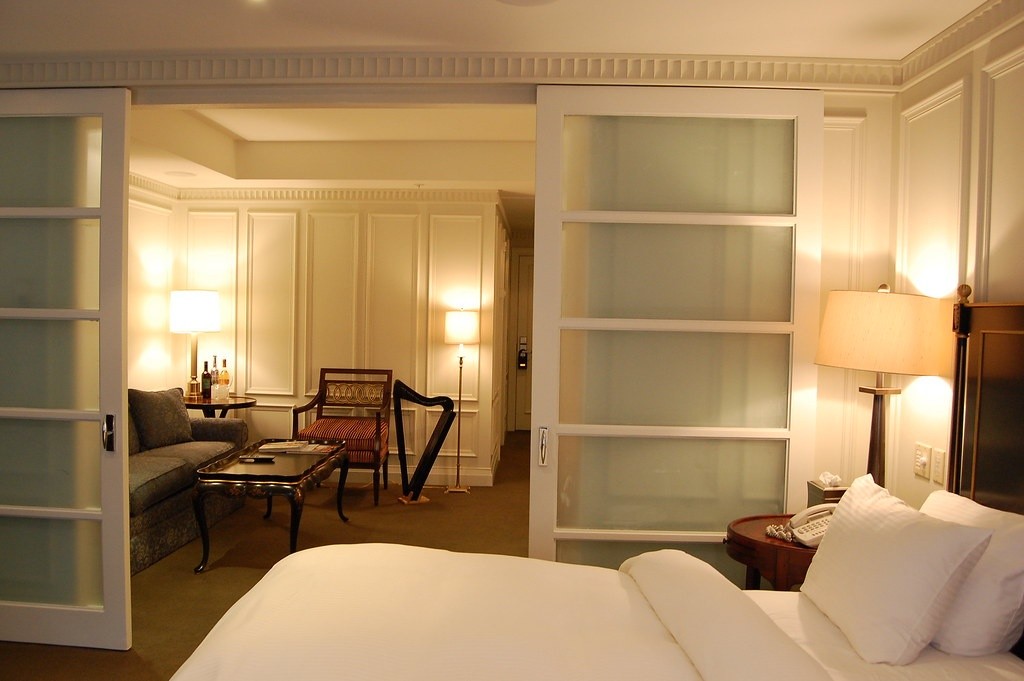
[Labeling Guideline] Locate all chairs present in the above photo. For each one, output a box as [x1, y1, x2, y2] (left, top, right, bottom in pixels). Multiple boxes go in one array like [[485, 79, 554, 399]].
[[293, 367, 393, 506]]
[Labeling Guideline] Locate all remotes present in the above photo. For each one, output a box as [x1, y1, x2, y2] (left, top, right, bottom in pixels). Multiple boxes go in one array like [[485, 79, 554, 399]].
[[239, 454, 275, 463]]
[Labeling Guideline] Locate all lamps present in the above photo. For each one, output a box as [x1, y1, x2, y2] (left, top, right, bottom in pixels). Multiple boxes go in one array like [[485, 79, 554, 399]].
[[444, 308, 479, 495], [813, 284, 957, 491], [168, 289, 221, 397]]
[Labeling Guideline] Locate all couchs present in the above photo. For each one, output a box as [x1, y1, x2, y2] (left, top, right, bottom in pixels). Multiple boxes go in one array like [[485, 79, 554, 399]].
[[128, 404, 248, 577]]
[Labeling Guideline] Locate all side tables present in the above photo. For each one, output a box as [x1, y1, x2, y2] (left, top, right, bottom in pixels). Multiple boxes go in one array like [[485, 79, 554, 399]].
[[182, 396, 257, 418]]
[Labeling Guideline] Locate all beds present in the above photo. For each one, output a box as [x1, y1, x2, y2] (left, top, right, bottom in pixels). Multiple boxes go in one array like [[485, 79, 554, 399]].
[[169, 286, 1024, 680]]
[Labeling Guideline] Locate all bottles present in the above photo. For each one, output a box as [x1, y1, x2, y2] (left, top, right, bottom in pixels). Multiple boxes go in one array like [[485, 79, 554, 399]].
[[201, 361, 212, 399], [219, 359, 230, 385], [211, 356, 220, 385]]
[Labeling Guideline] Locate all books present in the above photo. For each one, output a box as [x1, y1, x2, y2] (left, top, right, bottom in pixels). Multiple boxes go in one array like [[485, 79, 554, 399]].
[[258, 441, 309, 452], [285, 442, 338, 456]]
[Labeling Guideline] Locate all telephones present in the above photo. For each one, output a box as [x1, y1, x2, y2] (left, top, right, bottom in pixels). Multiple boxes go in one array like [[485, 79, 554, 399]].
[[766, 502, 840, 548]]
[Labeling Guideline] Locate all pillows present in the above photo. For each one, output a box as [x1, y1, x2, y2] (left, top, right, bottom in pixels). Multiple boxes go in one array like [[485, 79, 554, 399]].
[[799, 473, 994, 666], [128, 387, 194, 447], [918, 490, 1024, 655]]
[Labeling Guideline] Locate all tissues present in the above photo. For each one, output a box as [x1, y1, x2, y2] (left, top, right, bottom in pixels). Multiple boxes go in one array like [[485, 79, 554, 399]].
[[807, 468, 849, 508]]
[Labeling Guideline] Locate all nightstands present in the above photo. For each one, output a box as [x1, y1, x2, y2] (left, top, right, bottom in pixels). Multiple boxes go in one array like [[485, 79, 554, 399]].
[[722, 513, 817, 590]]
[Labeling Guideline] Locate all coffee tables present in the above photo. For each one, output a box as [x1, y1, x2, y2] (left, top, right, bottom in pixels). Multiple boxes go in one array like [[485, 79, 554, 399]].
[[191, 438, 350, 577]]
